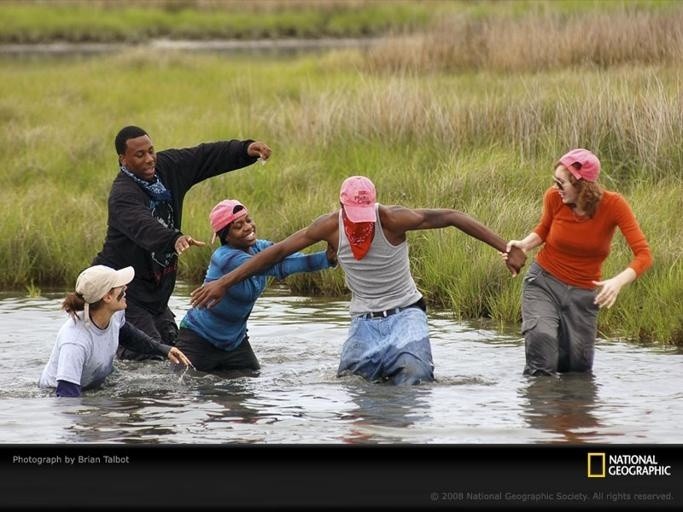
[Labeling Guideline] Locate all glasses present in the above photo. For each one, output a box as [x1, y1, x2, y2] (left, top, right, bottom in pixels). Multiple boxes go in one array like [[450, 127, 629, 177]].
[[552, 177, 573, 191]]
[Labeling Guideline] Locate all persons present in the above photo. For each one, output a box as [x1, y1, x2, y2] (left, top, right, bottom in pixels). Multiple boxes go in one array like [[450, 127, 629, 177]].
[[161, 200, 341, 382], [496, 148, 653, 374], [190, 176, 527, 386], [87, 125, 271, 358], [34, 265, 193, 396]]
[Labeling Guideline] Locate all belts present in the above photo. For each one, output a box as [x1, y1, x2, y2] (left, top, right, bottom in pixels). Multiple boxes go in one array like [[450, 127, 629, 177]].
[[357, 307, 405, 319]]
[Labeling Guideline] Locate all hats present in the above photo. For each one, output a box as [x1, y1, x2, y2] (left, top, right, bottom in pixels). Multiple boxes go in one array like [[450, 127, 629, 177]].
[[208, 199, 250, 245], [559, 148, 601, 183], [75, 265, 136, 304], [340, 176, 377, 223]]
